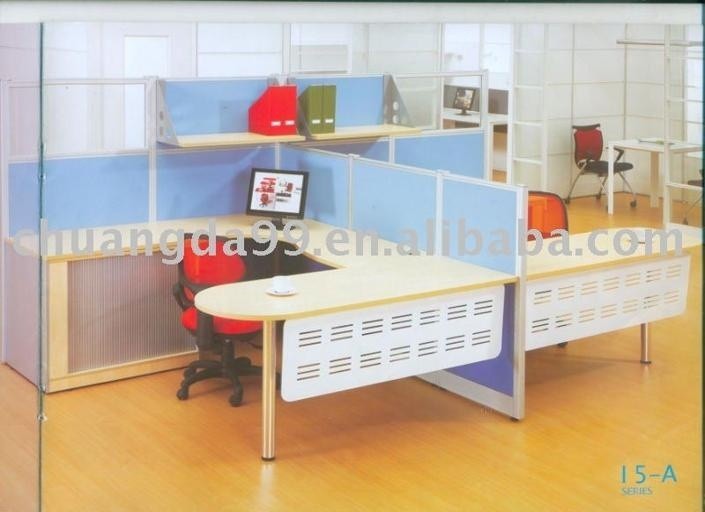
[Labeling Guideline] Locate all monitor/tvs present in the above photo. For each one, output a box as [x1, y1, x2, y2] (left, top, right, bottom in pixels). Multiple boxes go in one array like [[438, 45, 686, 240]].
[[452, 87, 476, 116], [245, 167, 309, 230]]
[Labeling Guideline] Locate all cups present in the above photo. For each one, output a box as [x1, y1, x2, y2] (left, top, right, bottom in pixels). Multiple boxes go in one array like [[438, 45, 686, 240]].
[[271, 275, 291, 292]]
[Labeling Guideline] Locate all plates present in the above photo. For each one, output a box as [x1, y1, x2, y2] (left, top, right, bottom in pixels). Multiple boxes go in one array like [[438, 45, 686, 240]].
[[266, 288, 298, 296]]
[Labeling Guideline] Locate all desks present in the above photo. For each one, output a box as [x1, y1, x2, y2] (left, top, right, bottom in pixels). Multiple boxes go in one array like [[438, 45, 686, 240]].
[[5, 213, 518, 461], [526, 223, 703, 364], [607, 139, 703, 216], [444, 108, 508, 128]]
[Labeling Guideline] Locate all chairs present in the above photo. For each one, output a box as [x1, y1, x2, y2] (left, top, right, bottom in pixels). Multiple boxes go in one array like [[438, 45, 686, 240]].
[[172, 233, 281, 408], [528, 190, 568, 348], [565, 123, 637, 212]]
